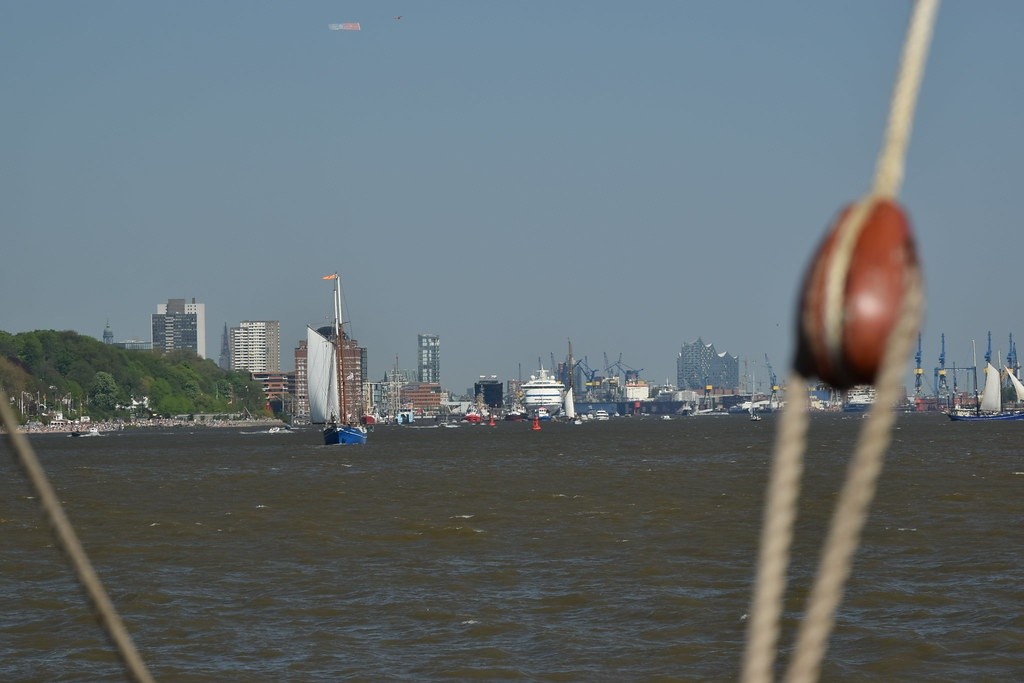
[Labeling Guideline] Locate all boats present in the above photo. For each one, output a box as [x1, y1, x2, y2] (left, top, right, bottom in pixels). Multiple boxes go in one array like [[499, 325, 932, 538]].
[[519, 369, 565, 417], [588, 414, 593, 420], [89, 428, 97, 435], [652, 376, 699, 414], [533, 408, 551, 422], [844, 395, 875, 412], [270, 427, 288, 433], [596, 410, 609, 421], [360, 407, 379, 425], [465, 408, 484, 423], [662, 416, 671, 420], [71, 432, 81, 435], [581, 415, 588, 421], [505, 407, 529, 423], [575, 419, 581, 424]]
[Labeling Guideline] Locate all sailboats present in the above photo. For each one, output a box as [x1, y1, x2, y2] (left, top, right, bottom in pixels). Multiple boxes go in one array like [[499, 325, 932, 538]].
[[321, 270, 367, 447], [946, 350, 1024, 421], [749, 361, 761, 421]]
